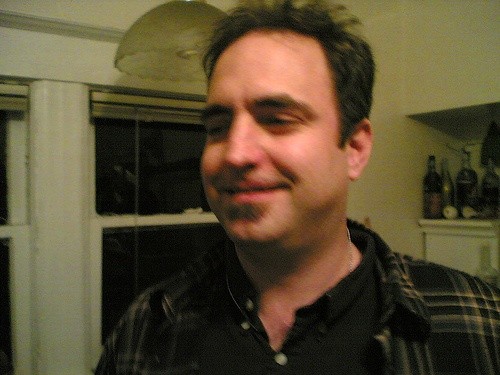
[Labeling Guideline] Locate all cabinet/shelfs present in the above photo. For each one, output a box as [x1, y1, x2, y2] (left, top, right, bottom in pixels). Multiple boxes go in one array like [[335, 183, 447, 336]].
[[418, 219, 500, 275]]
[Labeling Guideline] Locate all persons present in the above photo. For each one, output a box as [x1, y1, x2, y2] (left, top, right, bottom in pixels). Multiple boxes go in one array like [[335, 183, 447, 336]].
[[93, 0, 500, 375]]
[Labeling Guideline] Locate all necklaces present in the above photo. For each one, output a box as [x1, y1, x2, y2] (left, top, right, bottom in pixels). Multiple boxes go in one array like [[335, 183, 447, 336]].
[[345, 226, 354, 277]]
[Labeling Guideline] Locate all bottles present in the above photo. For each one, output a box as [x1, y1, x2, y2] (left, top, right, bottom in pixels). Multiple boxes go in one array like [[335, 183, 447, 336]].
[[422, 155, 442, 220], [442, 158, 458, 220], [476, 245, 500, 289], [479, 156, 500, 219], [455, 151, 479, 220]]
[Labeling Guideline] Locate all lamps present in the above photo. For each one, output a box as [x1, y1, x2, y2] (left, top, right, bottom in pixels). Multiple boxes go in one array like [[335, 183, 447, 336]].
[[113, 0, 228, 82]]
